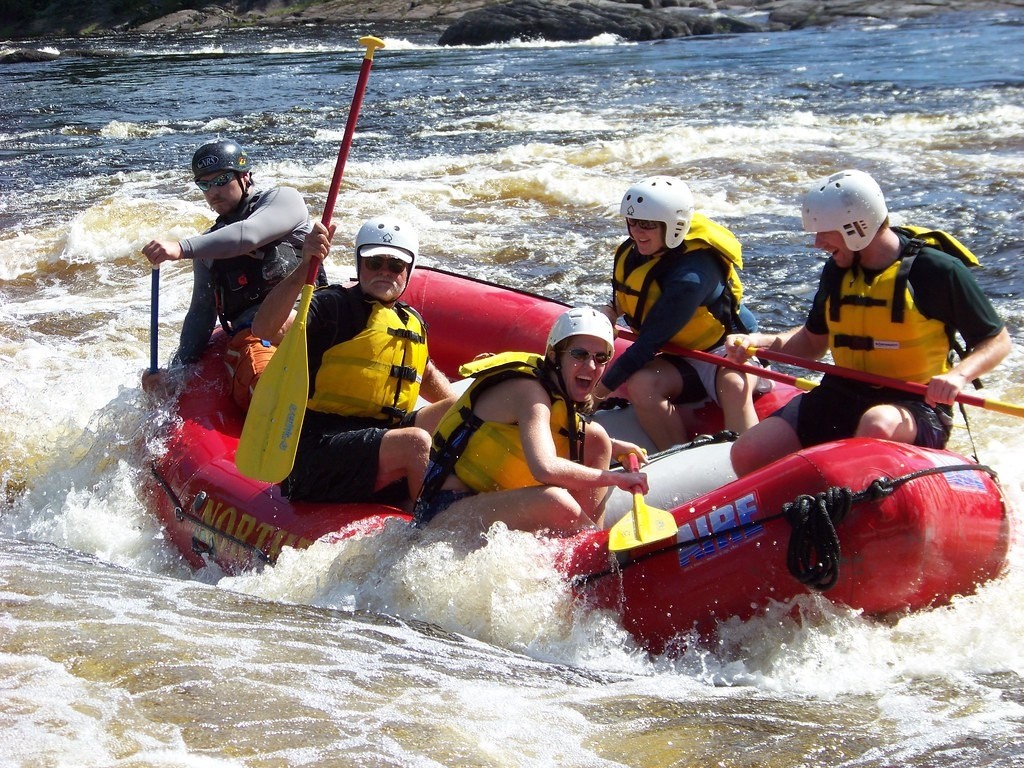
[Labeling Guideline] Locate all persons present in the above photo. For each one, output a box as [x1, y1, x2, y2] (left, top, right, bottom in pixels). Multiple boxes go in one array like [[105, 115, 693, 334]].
[[586, 175, 774, 451], [250, 215, 459, 512], [723, 169, 1012, 479], [141, 141, 329, 412], [412, 307, 647, 538]]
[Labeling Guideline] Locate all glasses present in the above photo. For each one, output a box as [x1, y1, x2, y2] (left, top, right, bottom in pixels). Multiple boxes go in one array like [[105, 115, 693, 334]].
[[628, 217, 660, 230], [362, 255, 406, 274], [196, 170, 235, 191], [557, 348, 612, 365]]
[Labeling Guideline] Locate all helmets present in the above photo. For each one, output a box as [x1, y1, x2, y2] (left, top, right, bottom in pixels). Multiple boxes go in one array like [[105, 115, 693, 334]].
[[619, 175, 694, 249], [802, 169, 889, 252], [355, 214, 419, 286], [545, 307, 615, 365], [191, 141, 252, 182]]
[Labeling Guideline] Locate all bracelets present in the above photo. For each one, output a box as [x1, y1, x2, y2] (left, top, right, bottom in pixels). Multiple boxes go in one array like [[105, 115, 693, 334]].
[[593, 394, 605, 401]]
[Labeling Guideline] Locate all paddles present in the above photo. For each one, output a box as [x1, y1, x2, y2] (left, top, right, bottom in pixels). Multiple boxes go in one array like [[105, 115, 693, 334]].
[[149, 261, 160, 374], [607, 447, 680, 552], [614, 327, 972, 429], [234, 34, 386, 483], [734, 338, 1024, 419]]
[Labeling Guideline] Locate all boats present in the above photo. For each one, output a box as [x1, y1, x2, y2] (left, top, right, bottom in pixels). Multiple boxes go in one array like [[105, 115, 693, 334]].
[[134, 266, 1013, 658]]
